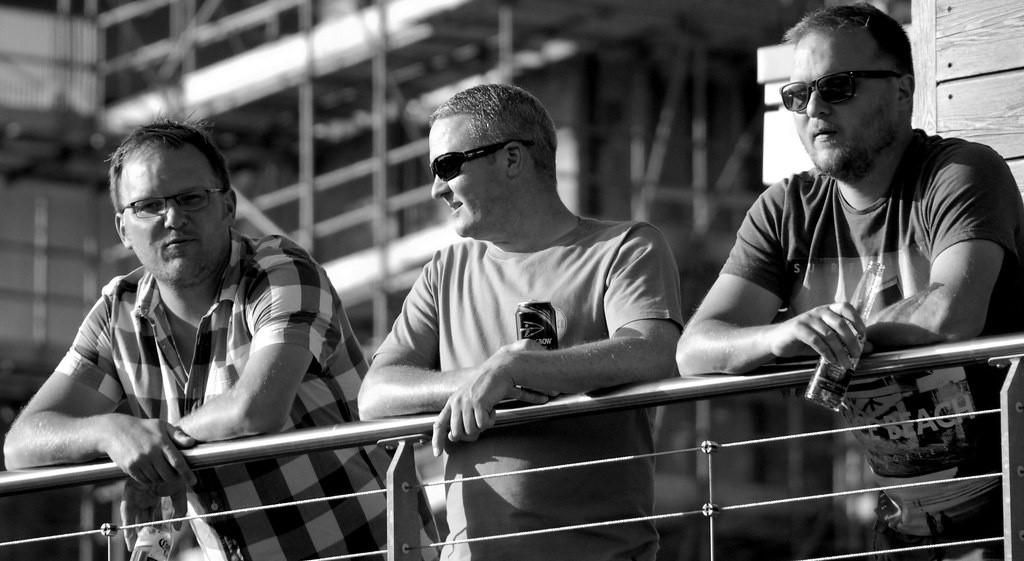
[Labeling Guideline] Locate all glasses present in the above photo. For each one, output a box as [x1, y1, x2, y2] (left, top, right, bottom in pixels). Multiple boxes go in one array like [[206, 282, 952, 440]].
[[430, 139, 537, 181], [118, 188, 225, 218], [780, 69, 901, 111]]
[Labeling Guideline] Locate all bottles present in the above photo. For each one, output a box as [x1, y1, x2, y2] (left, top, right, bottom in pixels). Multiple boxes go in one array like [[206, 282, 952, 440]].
[[128, 496, 174, 561], [805, 260, 885, 413]]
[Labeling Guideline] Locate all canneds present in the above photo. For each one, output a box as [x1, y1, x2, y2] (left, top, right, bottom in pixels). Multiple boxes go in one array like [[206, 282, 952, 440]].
[[515, 299, 560, 352]]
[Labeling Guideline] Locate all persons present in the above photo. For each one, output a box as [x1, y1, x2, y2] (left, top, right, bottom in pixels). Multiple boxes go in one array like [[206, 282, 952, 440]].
[[3, 118, 445, 561], [672, 0, 1023, 560], [357, 82, 686, 561]]
